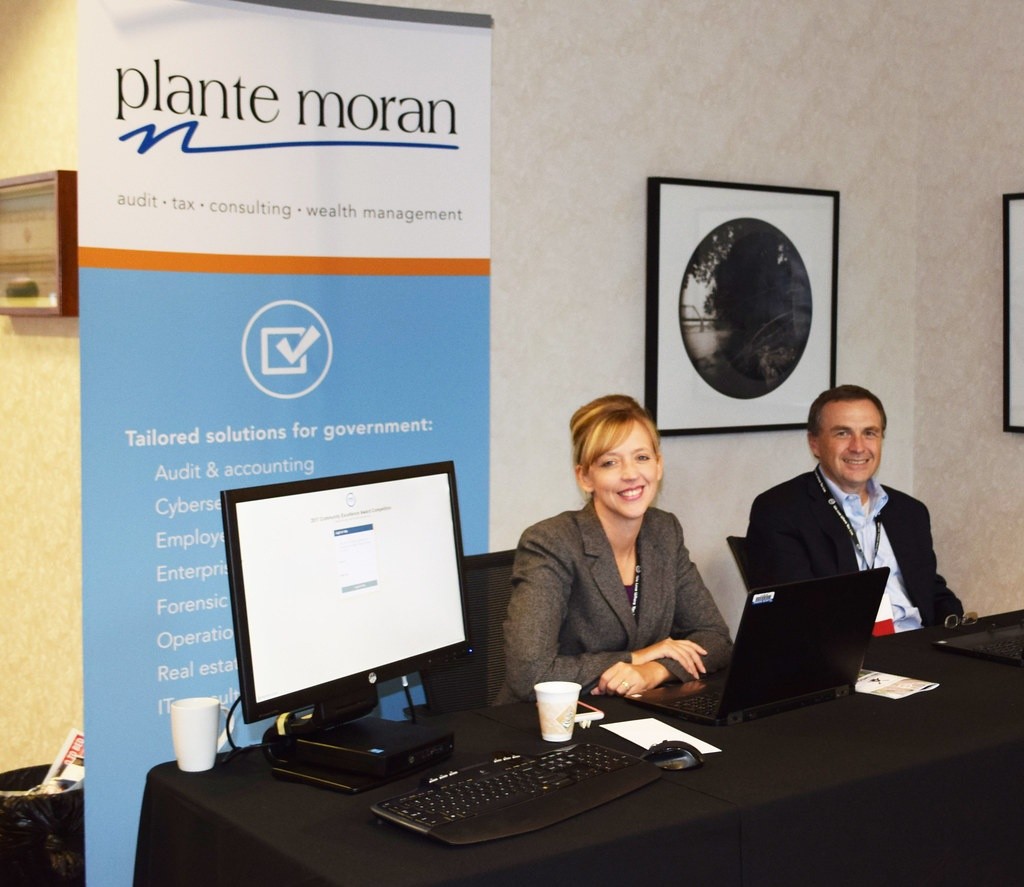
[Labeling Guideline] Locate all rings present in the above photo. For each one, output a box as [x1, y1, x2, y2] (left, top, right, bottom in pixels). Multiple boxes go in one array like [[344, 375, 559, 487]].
[[623, 681, 630, 690]]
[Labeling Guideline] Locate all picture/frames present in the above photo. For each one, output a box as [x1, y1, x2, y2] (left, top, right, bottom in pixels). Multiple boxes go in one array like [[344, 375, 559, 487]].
[[644, 177, 839, 433], [1002, 193, 1024, 434]]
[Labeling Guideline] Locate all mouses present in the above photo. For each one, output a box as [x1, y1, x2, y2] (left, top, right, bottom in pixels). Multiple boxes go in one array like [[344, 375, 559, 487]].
[[640, 739, 705, 771]]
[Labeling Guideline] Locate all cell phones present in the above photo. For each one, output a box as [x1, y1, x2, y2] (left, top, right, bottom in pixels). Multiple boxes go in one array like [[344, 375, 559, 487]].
[[573, 700, 605, 729]]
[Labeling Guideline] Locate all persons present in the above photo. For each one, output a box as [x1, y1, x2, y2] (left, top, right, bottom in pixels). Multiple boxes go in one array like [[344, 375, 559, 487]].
[[743, 383, 966, 638], [501, 394, 738, 702]]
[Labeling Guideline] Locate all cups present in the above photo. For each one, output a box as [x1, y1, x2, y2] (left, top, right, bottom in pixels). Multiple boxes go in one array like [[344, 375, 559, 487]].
[[534, 680, 582, 742], [171, 697, 235, 773]]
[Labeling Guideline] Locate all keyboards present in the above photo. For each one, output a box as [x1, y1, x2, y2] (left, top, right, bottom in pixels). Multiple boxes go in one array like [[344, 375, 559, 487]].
[[369, 742, 663, 845]]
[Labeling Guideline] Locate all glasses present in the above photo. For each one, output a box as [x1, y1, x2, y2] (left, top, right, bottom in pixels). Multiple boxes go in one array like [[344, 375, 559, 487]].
[[944, 611, 979, 628]]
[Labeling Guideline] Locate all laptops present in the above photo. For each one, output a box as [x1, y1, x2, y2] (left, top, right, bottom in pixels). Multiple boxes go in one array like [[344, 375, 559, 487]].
[[623, 566, 891, 727], [929, 618, 1024, 669]]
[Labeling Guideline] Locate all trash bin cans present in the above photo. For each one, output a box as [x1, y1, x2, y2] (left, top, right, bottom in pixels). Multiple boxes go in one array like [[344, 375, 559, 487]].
[[0, 762, 85, 887]]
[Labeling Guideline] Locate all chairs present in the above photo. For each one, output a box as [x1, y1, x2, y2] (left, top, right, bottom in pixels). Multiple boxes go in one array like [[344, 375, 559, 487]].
[[402, 551, 516, 720]]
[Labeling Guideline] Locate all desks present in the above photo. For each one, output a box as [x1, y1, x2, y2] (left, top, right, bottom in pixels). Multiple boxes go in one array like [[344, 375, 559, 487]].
[[131, 613, 1024, 887]]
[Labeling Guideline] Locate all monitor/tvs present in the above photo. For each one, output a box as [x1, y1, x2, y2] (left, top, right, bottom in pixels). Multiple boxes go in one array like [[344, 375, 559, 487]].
[[218, 460, 476, 793]]
